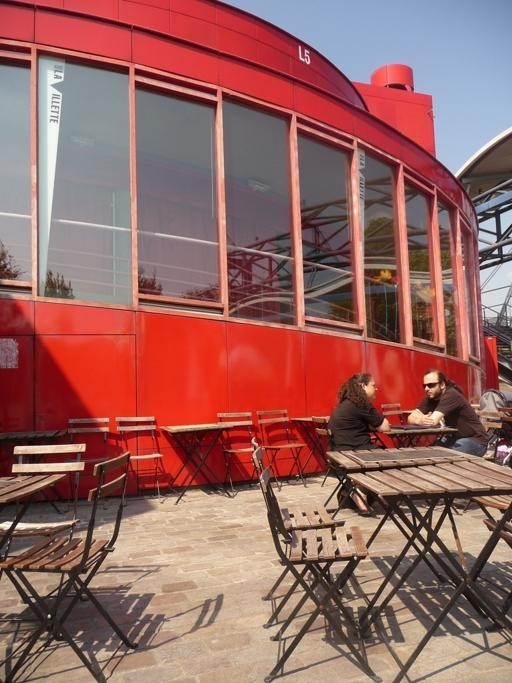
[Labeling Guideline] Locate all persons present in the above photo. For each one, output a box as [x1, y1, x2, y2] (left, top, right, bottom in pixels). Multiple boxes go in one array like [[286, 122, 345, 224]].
[[407, 368, 490, 508], [325, 372, 391, 516]]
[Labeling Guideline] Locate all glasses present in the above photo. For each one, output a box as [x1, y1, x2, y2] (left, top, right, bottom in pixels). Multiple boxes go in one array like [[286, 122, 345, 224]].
[[422, 381, 442, 389]]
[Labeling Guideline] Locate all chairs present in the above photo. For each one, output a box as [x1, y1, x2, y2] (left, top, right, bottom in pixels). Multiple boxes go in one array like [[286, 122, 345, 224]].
[[216, 408, 345, 498], [250, 437, 383, 682], [0, 403, 186, 683], [477, 406, 511, 459], [381, 403, 404, 427]]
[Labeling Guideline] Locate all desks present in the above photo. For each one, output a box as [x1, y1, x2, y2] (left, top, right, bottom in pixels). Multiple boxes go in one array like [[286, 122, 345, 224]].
[[384, 427, 457, 447], [159, 422, 233, 505], [325, 445, 512, 683]]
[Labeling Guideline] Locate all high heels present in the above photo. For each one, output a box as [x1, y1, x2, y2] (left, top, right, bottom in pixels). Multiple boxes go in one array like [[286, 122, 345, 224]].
[[337, 490, 379, 516]]
